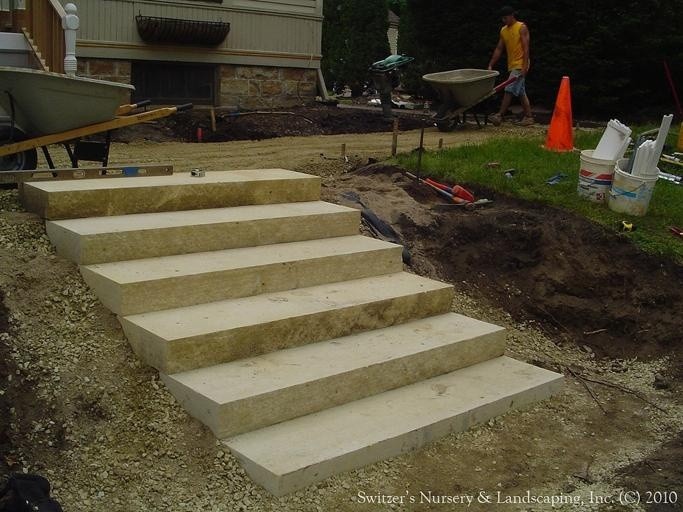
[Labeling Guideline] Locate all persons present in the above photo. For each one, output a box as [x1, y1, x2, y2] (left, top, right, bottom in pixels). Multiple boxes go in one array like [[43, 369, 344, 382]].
[[487, 5, 536, 127]]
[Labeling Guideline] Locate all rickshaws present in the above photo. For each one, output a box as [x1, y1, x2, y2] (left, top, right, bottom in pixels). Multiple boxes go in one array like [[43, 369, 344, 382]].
[[421, 68, 523, 134], [0, 64, 195, 191]]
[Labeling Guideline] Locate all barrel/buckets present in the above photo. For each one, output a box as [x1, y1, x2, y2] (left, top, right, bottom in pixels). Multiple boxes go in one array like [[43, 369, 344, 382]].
[[607, 157, 660, 218], [576, 149, 623, 205]]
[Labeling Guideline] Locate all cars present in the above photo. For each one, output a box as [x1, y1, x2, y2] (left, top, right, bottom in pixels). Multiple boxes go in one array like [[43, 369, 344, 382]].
[[366, 93, 415, 110]]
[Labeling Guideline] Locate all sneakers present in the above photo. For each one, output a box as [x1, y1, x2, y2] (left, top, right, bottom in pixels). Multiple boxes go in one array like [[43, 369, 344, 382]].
[[518, 116, 534, 126], [488, 113, 502, 126]]
